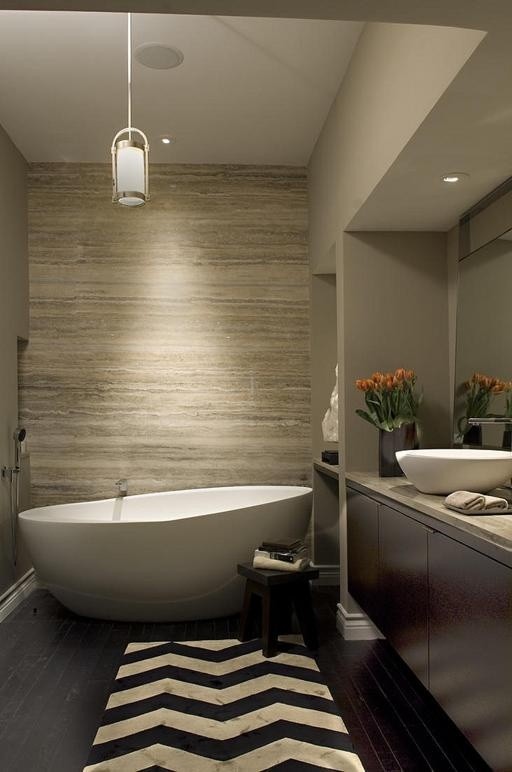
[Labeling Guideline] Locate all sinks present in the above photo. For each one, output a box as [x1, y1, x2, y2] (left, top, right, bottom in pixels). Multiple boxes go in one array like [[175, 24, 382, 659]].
[[395, 449, 512, 496]]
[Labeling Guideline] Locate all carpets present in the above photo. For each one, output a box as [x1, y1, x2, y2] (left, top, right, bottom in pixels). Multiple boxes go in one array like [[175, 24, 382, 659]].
[[82, 633, 366, 772]]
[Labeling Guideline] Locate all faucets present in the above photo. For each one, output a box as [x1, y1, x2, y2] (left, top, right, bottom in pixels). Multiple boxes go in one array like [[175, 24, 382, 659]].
[[467, 417, 512, 426], [116, 477, 128, 495]]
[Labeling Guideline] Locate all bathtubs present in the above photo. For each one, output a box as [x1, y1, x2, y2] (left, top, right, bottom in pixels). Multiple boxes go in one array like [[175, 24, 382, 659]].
[[17, 484, 313, 622]]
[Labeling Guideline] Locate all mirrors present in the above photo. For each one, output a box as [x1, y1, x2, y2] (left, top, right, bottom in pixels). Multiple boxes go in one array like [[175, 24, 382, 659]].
[[451, 229, 512, 451]]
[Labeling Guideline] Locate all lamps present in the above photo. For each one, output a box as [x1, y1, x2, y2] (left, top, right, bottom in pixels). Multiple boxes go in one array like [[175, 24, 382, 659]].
[[110, 12, 150, 207]]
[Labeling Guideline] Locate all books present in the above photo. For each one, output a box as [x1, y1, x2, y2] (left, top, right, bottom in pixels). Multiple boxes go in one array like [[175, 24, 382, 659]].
[[254, 549, 309, 563], [262, 536, 304, 549], [258, 546, 306, 553]]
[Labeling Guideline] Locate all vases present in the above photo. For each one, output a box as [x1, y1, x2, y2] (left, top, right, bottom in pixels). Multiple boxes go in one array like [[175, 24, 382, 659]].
[[380, 421, 417, 477], [463, 424, 481, 445]]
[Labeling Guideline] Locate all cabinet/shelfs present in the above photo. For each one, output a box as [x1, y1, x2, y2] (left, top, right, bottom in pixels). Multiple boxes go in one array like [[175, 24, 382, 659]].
[[378, 505, 430, 690], [428, 527, 512, 771], [346, 487, 378, 625]]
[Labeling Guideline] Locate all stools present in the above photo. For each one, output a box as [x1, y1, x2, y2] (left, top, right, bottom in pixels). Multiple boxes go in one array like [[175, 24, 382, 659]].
[[238, 560, 320, 658]]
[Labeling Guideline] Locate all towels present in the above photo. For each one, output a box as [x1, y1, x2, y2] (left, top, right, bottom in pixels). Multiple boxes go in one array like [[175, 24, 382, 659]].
[[483, 495, 508, 510], [253, 539, 311, 573], [443, 490, 486, 511]]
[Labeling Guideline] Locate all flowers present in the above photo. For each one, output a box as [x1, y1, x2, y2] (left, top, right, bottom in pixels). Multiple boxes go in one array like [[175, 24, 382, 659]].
[[457, 371, 512, 434], [354, 367, 425, 431]]
